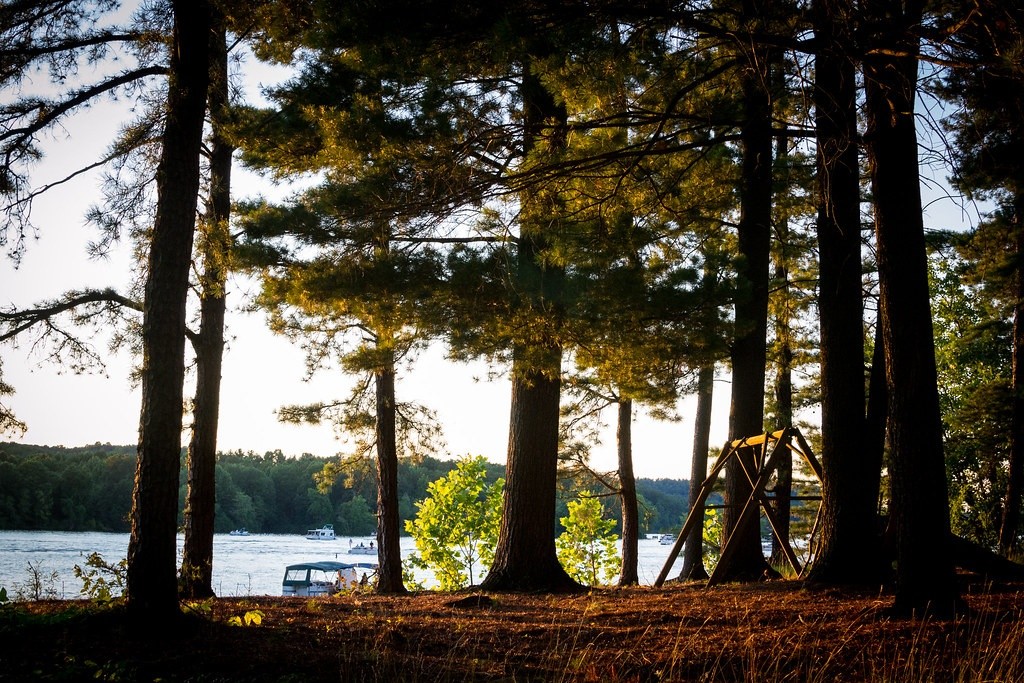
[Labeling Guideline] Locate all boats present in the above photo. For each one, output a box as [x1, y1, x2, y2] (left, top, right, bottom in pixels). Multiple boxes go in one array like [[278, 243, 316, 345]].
[[279, 559, 378, 598], [348, 539, 378, 555], [230, 530, 250, 536], [305, 529, 335, 541]]
